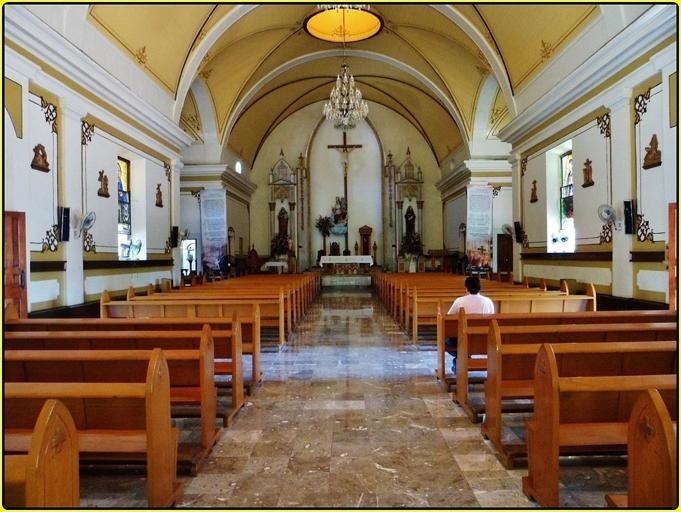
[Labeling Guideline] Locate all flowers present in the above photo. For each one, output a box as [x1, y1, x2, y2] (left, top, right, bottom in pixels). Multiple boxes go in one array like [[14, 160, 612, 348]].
[[315, 216, 334, 237], [270, 235, 289, 255], [397, 230, 426, 272]]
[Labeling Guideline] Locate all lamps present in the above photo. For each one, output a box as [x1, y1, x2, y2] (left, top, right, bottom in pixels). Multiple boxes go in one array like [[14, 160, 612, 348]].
[[228, 226, 235, 237], [459, 223, 466, 232], [303, 4, 384, 131]]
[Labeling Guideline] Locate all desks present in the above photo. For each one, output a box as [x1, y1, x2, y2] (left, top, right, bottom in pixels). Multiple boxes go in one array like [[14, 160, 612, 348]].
[[318, 255, 374, 274]]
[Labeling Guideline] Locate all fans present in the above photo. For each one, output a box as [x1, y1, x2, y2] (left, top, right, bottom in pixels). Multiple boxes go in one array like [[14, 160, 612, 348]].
[[219, 255, 238, 273], [131, 240, 142, 260], [120, 238, 132, 260], [502, 224, 516, 239], [74, 210, 96, 239], [597, 204, 618, 231]]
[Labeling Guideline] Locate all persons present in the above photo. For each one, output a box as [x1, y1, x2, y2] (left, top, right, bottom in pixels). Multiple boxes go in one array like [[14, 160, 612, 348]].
[[444, 276, 495, 375]]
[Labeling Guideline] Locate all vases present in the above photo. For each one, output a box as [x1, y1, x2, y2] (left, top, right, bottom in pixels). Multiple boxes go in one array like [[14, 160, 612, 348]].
[[275, 254, 288, 262]]
